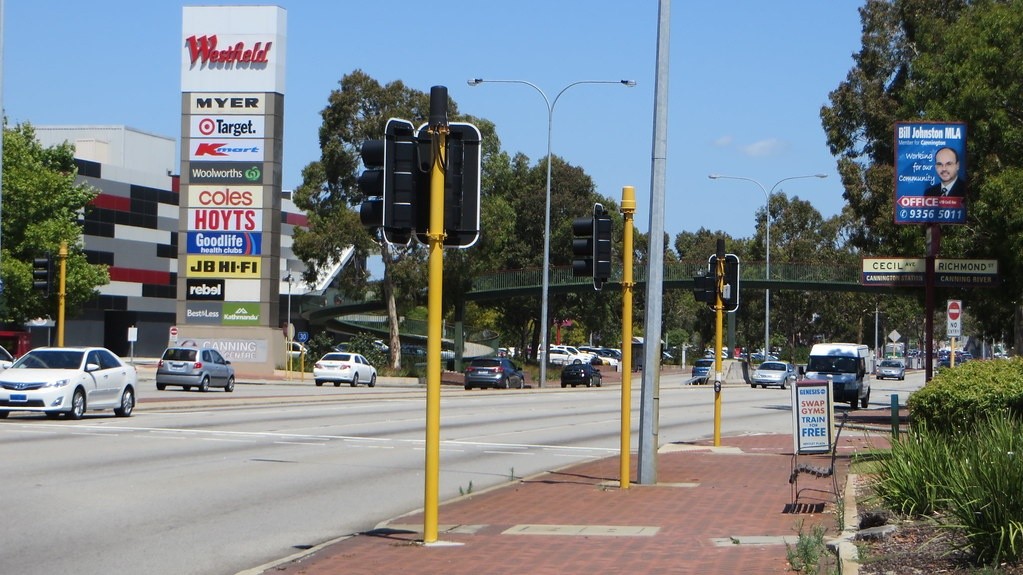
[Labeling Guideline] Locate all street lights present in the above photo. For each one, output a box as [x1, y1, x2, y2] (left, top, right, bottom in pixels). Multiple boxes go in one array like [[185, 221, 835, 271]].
[[467, 78, 637, 386], [709, 172, 829, 361], [282, 274, 295, 361]]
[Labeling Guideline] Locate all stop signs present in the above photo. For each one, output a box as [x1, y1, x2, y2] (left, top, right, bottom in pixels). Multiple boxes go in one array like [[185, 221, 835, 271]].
[[948, 302, 959, 320], [170, 328, 177, 335]]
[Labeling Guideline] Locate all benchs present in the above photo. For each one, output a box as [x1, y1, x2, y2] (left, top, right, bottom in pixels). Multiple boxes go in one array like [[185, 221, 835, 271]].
[[789, 411, 849, 511]]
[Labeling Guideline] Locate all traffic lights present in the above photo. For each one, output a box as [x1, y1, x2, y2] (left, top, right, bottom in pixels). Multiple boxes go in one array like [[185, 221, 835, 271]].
[[572, 202, 611, 291], [360, 118, 416, 247], [33, 253, 53, 298]]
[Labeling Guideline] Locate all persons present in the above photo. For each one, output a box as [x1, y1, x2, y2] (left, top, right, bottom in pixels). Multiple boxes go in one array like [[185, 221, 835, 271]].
[[923, 146, 966, 199]]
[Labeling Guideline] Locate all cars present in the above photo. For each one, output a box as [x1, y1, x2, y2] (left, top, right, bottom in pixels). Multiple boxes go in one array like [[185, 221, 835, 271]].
[[750, 362, 798, 389], [884, 343, 1008, 369], [0, 346, 137, 421], [692, 346, 782, 383], [497, 341, 675, 366], [287, 340, 425, 360], [465, 359, 524, 389], [156, 347, 236, 392], [561, 363, 602, 388], [313, 352, 377, 387], [876, 359, 905, 381]]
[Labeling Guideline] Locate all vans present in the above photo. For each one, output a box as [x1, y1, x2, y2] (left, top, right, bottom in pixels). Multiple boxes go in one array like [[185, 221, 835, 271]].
[[797, 342, 871, 411]]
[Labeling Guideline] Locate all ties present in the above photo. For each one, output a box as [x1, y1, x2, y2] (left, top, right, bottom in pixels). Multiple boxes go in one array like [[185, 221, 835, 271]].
[[940, 188, 947, 196]]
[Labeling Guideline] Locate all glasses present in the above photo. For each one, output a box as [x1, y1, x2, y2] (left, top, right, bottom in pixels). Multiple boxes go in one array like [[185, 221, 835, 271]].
[[936, 162, 957, 168]]
